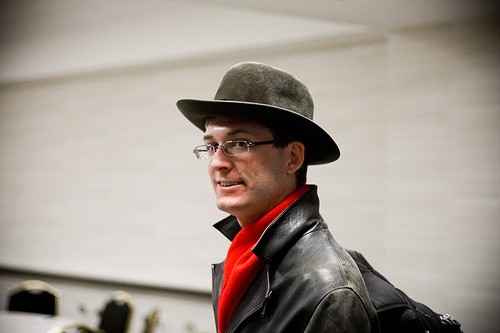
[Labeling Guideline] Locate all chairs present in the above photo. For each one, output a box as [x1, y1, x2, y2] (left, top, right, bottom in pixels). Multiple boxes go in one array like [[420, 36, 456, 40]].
[[4, 280, 159, 333]]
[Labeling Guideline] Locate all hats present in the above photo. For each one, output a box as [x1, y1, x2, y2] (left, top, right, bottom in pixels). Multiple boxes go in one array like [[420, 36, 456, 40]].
[[176, 62, 341, 164]]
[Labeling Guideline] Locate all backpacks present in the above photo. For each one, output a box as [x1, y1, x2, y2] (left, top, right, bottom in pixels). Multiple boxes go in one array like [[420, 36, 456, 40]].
[[345, 248, 465, 333]]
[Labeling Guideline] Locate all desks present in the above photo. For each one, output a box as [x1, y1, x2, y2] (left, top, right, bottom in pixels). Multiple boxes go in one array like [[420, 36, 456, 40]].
[[0, 309, 84, 333]]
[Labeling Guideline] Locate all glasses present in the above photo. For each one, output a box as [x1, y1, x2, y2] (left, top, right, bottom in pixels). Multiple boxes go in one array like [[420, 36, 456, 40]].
[[194, 137, 292, 159]]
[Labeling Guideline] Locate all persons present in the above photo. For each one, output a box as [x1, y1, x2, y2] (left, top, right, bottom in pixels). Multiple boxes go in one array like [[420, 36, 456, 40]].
[[176, 62, 379, 333]]
